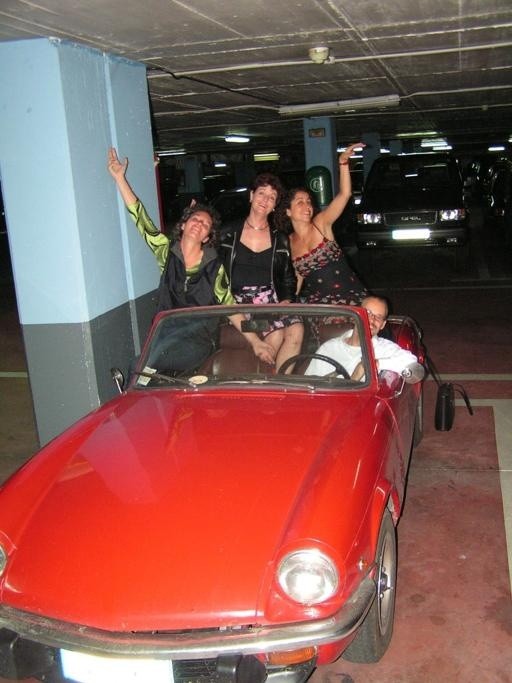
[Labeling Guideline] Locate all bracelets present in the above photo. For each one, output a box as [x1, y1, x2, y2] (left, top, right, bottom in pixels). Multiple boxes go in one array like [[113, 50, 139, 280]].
[[338, 161, 348, 165]]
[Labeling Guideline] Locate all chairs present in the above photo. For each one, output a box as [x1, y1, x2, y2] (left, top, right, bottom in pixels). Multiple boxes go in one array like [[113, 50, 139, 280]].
[[197, 323, 356, 378]]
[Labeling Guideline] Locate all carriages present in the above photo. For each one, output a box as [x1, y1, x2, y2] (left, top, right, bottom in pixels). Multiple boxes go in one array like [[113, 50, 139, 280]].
[[1, 303, 424, 683]]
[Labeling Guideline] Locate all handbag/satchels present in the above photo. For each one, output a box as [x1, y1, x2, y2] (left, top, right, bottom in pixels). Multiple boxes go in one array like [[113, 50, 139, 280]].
[[425, 355, 474, 432], [232, 284, 274, 306]]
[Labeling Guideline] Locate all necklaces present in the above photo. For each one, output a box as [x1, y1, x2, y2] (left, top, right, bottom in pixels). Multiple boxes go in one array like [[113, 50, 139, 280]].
[[246, 218, 269, 231]]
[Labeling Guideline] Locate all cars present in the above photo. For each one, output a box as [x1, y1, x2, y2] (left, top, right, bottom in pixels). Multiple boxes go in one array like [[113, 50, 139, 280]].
[[164, 186, 250, 246], [352, 152, 512, 258]]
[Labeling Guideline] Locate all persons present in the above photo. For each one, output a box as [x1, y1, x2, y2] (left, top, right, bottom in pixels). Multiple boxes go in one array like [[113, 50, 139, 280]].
[[183, 174, 323, 376], [304, 295, 417, 386], [285, 144, 369, 323], [108, 147, 277, 383]]
